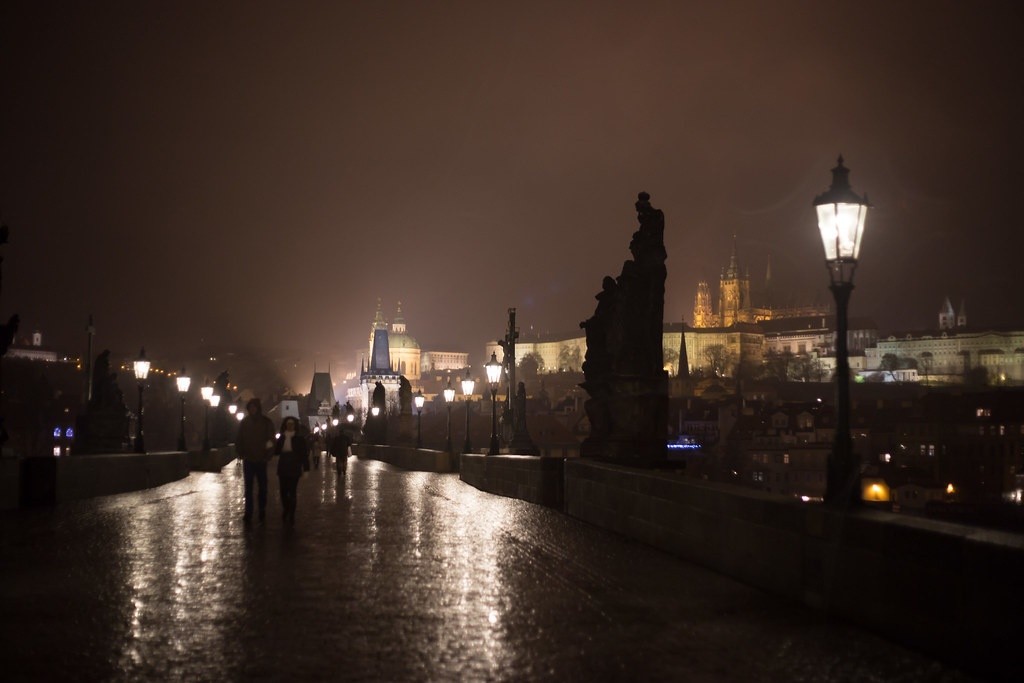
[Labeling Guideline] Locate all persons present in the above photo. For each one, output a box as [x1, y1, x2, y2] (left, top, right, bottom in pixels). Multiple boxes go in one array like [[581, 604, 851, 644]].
[[312, 433, 323, 468], [236, 398, 279, 521], [269, 416, 311, 533], [330, 429, 350, 476]]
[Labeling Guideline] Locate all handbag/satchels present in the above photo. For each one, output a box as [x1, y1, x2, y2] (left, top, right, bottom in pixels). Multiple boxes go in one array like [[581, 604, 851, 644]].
[[348, 446, 352, 457]]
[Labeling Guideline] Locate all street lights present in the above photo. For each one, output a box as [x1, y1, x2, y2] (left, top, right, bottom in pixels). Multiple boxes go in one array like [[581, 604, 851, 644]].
[[202, 377, 214, 451], [443, 380, 456, 450], [414, 389, 426, 449], [372, 404, 380, 443], [813, 156, 872, 509], [486, 352, 504, 456], [461, 367, 476, 454], [209, 393, 220, 450], [175, 367, 191, 450], [133, 347, 150, 453]]
